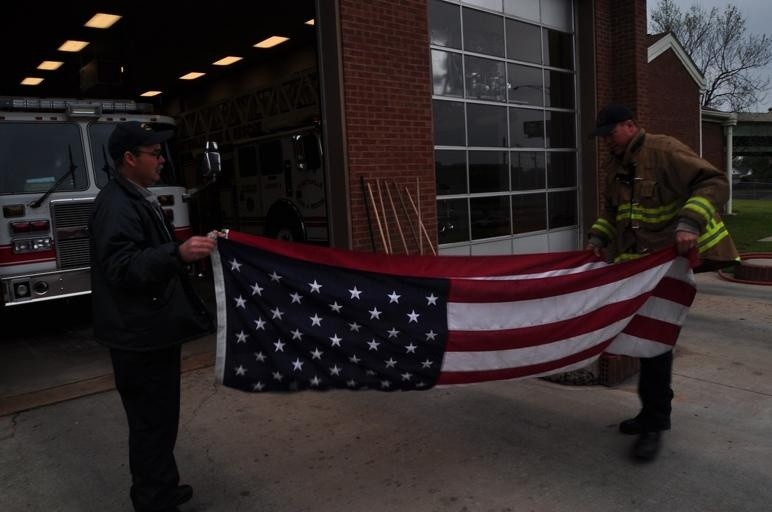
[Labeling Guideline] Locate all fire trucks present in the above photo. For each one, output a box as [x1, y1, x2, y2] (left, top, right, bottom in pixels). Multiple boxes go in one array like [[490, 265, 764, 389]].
[[175, 118, 330, 247], [1, 92, 225, 343]]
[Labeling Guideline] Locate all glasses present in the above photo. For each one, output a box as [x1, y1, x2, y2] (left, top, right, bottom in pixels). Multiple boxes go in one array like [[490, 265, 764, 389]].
[[128, 148, 164, 160]]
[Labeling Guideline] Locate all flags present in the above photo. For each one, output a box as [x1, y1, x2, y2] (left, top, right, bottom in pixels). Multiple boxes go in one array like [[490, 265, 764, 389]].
[[206, 224, 700, 394]]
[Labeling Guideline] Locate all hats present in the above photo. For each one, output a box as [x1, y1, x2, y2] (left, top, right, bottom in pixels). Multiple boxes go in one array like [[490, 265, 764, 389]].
[[592, 103, 633, 136], [107, 120, 175, 161]]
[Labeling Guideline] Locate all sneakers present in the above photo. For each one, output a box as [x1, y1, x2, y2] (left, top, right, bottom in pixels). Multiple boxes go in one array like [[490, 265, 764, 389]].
[[129, 480, 194, 511], [619, 405, 672, 464]]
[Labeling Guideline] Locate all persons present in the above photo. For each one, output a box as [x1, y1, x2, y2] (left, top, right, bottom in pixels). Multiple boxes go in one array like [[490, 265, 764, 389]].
[[88, 122, 225, 512], [584, 99, 730, 462]]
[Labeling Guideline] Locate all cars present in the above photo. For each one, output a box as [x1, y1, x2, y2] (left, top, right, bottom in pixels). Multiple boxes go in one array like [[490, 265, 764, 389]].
[[473, 213, 510, 230]]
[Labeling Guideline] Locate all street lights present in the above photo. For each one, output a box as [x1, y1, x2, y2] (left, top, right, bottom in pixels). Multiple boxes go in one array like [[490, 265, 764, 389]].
[[513, 83, 552, 98]]
